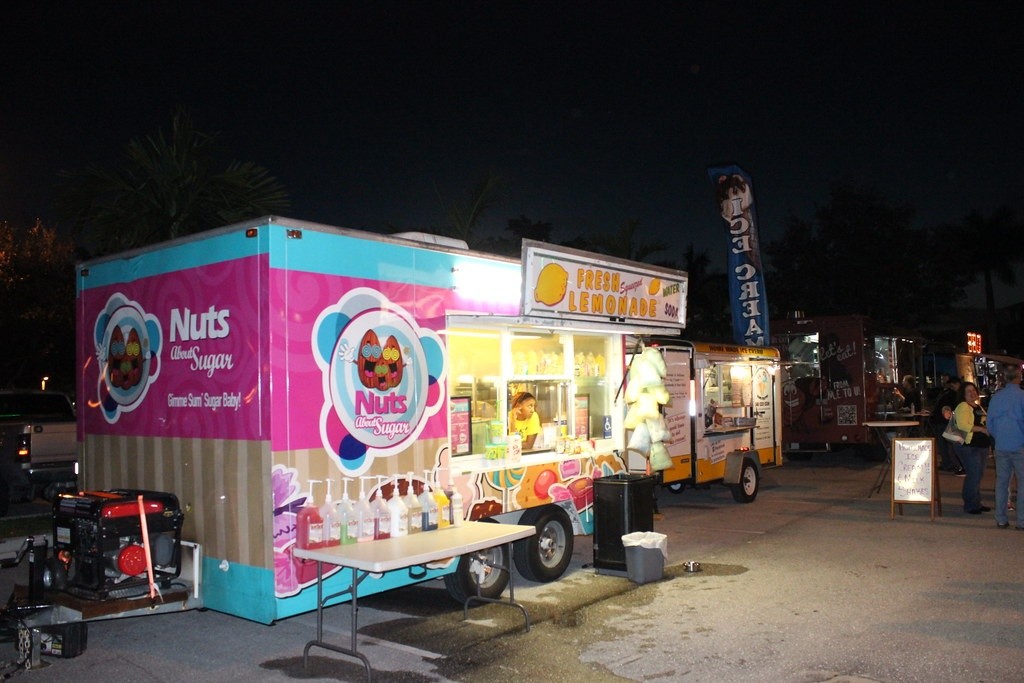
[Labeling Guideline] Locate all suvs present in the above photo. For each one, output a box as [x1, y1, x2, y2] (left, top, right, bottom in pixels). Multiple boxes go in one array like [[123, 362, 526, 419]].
[[1, 389, 79, 483]]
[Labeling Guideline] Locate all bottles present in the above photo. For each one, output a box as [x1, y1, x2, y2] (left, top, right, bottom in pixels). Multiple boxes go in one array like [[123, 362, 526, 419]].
[[911, 403, 915, 414]]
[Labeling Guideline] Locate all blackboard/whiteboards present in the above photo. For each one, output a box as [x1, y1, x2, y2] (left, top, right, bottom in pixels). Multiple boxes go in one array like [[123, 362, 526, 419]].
[[891, 437, 940, 504]]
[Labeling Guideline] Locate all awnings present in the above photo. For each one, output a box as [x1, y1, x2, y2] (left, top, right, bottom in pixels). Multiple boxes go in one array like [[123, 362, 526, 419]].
[[443, 309, 680, 405], [702, 360, 813, 389], [873, 333, 929, 373], [978, 354, 1024, 365]]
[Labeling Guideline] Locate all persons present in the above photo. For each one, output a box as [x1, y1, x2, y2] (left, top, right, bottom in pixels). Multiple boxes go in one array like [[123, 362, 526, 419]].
[[893, 374, 922, 438], [505, 392, 542, 451], [933, 373, 961, 471], [943, 383, 992, 514], [942, 406, 966, 475], [986, 363, 1024, 532]]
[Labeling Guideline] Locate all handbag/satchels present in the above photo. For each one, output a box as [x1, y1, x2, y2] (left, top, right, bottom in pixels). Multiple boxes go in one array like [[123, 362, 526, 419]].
[[942, 412, 967, 445]]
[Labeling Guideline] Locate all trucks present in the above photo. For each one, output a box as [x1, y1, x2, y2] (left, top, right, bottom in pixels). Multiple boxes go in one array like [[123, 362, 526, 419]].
[[770, 314, 1024, 464], [639, 337, 783, 503], [77, 215, 691, 627]]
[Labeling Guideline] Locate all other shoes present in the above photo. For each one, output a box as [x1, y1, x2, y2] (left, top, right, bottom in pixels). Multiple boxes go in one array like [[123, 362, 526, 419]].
[[997, 522, 1009, 528], [953, 468, 967, 477], [968, 510, 982, 515], [1007, 500, 1013, 511], [980, 506, 991, 512], [1016, 525, 1024, 530]]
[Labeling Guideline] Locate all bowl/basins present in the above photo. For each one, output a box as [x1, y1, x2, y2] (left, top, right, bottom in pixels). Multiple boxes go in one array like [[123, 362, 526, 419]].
[[683, 561, 701, 572]]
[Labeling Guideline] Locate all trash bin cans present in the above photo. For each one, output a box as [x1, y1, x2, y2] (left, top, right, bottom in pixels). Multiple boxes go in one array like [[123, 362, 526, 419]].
[[621, 531, 667, 583], [592, 473, 655, 571]]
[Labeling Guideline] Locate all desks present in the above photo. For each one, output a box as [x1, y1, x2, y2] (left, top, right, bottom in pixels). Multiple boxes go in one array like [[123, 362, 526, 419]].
[[861, 420, 920, 499], [871, 411, 932, 437], [292, 520, 537, 683]]
